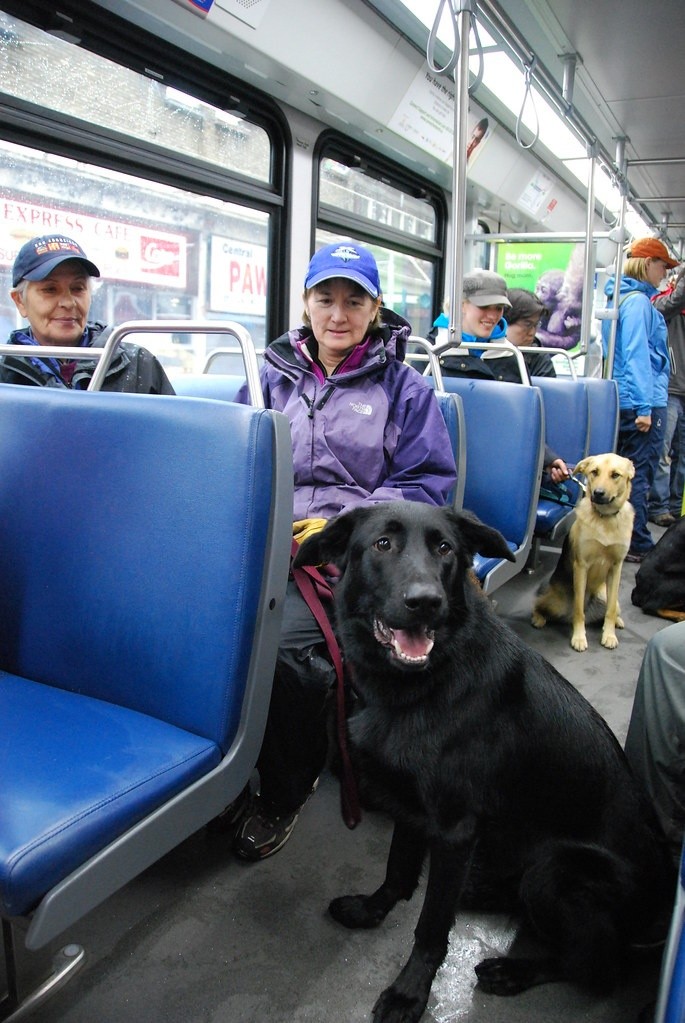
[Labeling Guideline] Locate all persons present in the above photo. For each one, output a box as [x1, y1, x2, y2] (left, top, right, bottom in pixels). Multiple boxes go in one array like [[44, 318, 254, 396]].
[[0, 234, 177, 395], [411, 266, 558, 386], [651, 269, 684, 526], [600, 237, 680, 562], [234, 241, 461, 864]]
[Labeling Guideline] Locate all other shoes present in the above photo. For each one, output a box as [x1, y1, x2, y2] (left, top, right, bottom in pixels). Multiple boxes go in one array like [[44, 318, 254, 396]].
[[624, 546, 651, 563], [648, 511, 675, 527], [644, 608, 685, 622]]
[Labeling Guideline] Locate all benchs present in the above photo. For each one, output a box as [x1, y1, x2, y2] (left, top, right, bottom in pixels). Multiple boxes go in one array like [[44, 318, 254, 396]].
[[0, 321, 621, 1022]]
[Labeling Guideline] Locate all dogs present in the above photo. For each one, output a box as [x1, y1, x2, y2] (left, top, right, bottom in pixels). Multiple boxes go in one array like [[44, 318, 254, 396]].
[[630, 514, 685, 624], [293, 499, 678, 1023], [530, 452, 636, 653]]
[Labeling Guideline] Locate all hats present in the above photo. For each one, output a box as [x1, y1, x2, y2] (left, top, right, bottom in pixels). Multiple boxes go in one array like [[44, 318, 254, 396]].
[[462, 269, 512, 308], [12, 234, 101, 288], [626, 237, 680, 269], [305, 242, 381, 298]]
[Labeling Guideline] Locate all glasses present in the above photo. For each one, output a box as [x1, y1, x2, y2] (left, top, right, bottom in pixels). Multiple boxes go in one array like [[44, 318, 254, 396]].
[[516, 320, 542, 329]]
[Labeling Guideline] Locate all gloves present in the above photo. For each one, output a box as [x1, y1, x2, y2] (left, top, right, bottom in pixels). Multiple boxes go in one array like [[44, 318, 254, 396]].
[[292, 517, 328, 546]]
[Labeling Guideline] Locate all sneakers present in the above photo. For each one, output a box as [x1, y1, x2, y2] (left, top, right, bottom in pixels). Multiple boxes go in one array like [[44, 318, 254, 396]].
[[224, 774, 320, 862]]
